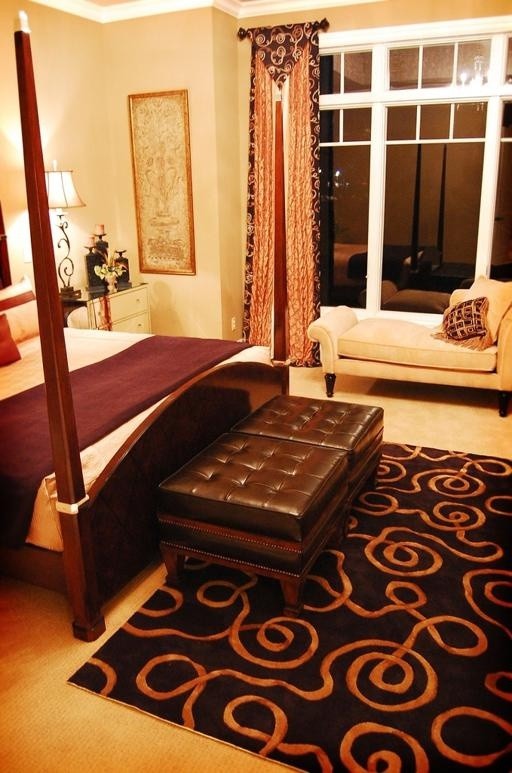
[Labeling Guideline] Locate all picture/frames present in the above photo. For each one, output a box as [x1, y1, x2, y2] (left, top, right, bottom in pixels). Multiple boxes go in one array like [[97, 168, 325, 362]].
[[128, 90, 196, 275]]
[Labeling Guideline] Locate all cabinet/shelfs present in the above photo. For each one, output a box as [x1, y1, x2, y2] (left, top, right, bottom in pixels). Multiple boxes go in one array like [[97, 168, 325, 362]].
[[62, 282, 151, 334]]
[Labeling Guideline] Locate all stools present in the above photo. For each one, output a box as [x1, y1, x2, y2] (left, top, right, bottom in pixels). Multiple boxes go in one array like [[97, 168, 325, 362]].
[[157, 432, 350, 619], [229, 395, 384, 542]]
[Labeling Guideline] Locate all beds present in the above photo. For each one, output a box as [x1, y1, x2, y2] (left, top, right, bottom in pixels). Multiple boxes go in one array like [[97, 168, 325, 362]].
[[0, 30, 290, 641]]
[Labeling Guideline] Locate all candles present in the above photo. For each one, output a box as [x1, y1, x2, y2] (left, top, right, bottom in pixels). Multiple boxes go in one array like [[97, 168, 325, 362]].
[[95, 223, 104, 235], [86, 236, 95, 247]]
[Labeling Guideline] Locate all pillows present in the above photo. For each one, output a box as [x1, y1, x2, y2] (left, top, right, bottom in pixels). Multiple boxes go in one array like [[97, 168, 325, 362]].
[[0, 313, 22, 367], [0, 275, 40, 344], [430, 296, 492, 351], [463, 275, 512, 342]]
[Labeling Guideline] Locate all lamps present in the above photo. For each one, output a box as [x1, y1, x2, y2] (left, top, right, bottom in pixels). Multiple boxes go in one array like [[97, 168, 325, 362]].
[[455, 44, 512, 111], [43, 160, 86, 293]]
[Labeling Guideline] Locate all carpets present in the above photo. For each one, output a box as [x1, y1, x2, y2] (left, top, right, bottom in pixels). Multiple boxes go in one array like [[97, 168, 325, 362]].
[[65, 444, 512, 772]]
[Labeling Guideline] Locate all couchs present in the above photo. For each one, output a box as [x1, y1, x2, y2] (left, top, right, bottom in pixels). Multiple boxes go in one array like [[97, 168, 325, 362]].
[[307, 306, 512, 417], [360, 279, 474, 314]]
